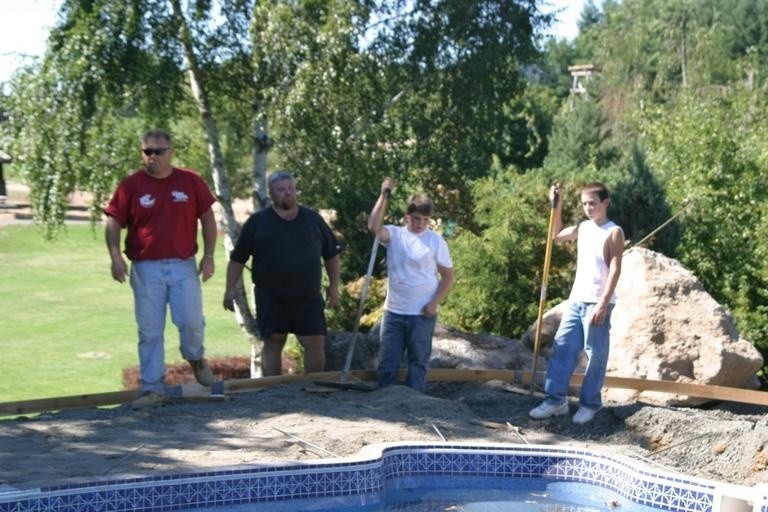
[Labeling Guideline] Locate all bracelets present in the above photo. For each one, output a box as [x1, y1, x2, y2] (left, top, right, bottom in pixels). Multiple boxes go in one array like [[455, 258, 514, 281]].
[[203, 253, 214, 259]]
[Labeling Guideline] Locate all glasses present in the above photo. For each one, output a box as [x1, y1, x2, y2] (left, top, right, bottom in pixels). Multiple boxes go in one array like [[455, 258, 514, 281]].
[[143, 147, 171, 155]]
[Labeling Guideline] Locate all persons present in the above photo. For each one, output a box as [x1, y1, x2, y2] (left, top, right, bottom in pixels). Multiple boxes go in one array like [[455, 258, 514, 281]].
[[526, 180, 625, 424], [103, 128, 217, 410], [366, 177, 456, 394], [222, 168, 342, 377]]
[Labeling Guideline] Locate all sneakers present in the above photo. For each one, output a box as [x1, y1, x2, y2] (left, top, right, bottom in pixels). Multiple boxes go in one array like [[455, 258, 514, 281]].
[[189, 358, 213, 386], [132, 390, 165, 409], [573, 406, 603, 423], [530, 400, 568, 419]]
